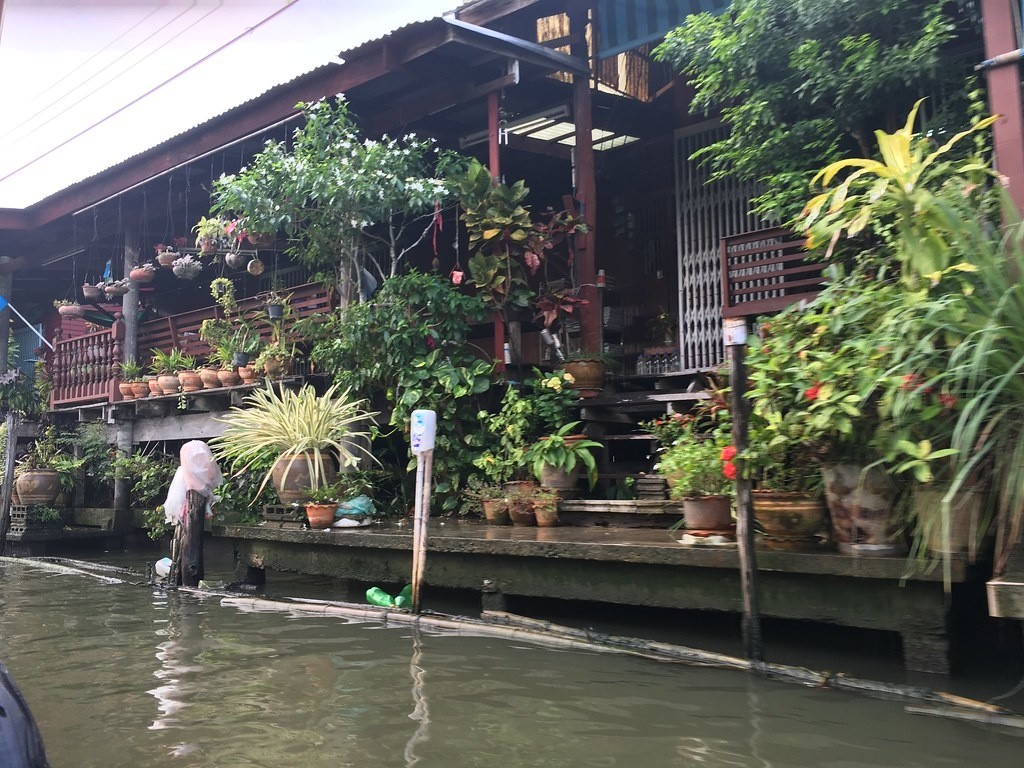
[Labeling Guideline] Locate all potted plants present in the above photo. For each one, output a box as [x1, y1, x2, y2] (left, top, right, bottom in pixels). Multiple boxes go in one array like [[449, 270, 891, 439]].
[[533, 487, 559, 527], [557, 352, 624, 387], [506, 488, 535, 527], [481, 486, 509, 524], [650, 305, 677, 346], [503, 454, 535, 490], [683, 444, 730, 528], [818, 400, 903, 542], [750, 446, 828, 538], [540, 434, 589, 492], [15, 221, 385, 527], [913, 407, 982, 548]]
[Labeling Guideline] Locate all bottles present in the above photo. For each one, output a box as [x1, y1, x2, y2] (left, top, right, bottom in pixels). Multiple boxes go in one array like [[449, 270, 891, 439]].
[[198, 579, 227, 590], [395, 583, 412, 608], [635, 349, 679, 375], [240, 584, 257, 591], [366, 587, 395, 610]]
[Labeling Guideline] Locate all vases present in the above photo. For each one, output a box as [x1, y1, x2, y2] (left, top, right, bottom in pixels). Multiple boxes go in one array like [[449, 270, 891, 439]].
[[667, 464, 685, 493]]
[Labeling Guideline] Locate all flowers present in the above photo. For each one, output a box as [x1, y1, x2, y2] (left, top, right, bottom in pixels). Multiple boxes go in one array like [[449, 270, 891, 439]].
[[638, 411, 698, 445], [535, 369, 583, 436]]
